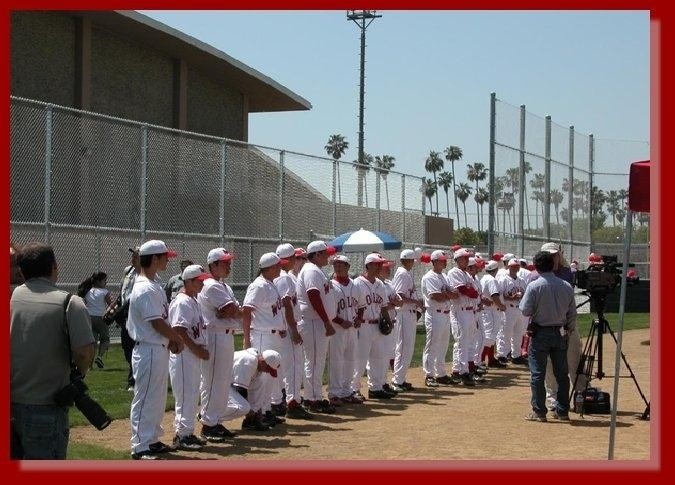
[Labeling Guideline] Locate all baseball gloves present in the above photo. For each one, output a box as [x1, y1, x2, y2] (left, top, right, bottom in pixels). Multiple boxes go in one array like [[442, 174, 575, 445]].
[[379, 317, 391, 335]]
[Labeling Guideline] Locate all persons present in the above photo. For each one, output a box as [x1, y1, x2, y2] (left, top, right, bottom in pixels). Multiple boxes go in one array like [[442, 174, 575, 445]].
[[541, 242, 592, 413], [589, 252, 601, 271], [9, 242, 539, 458], [627, 267, 636, 279], [519, 251, 577, 422], [570, 260, 578, 290]]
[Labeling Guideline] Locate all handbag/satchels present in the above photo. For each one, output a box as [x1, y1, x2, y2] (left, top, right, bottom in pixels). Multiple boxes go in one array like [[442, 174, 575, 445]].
[[575, 387, 610, 413]]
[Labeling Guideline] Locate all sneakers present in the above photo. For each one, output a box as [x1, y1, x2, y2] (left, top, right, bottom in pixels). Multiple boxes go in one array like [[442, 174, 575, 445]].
[[134, 435, 207, 460], [241, 405, 287, 431], [522, 411, 547, 422], [198, 412, 235, 443], [283, 394, 365, 420], [551, 414, 569, 420], [488, 356, 525, 368], [95, 358, 105, 369], [425, 365, 488, 387], [368, 382, 413, 398]]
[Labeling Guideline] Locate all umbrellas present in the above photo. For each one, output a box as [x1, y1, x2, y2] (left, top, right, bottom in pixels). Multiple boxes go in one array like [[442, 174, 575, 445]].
[[329, 227, 402, 274]]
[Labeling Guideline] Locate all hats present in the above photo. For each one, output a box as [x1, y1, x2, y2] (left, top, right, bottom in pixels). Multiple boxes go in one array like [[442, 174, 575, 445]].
[[129, 240, 177, 259], [502, 253, 527, 267], [329, 256, 351, 266], [262, 350, 280, 377], [453, 248, 486, 267], [430, 250, 448, 261], [541, 243, 562, 256], [306, 240, 328, 254], [207, 248, 235, 265], [258, 243, 307, 269], [182, 264, 208, 280], [365, 253, 394, 269], [484, 260, 498, 271], [399, 250, 417, 260]]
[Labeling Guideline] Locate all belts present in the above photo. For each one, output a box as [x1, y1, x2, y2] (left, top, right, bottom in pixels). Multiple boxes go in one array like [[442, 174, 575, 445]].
[[362, 320, 379, 324], [437, 310, 450, 314], [462, 307, 474, 311]]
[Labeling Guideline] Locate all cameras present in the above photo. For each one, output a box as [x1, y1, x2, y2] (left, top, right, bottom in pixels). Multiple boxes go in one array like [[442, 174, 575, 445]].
[[52, 376, 111, 431], [377, 318, 392, 335]]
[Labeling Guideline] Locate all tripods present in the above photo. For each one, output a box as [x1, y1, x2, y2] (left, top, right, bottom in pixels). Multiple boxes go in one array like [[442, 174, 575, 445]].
[[568, 316, 649, 419]]
